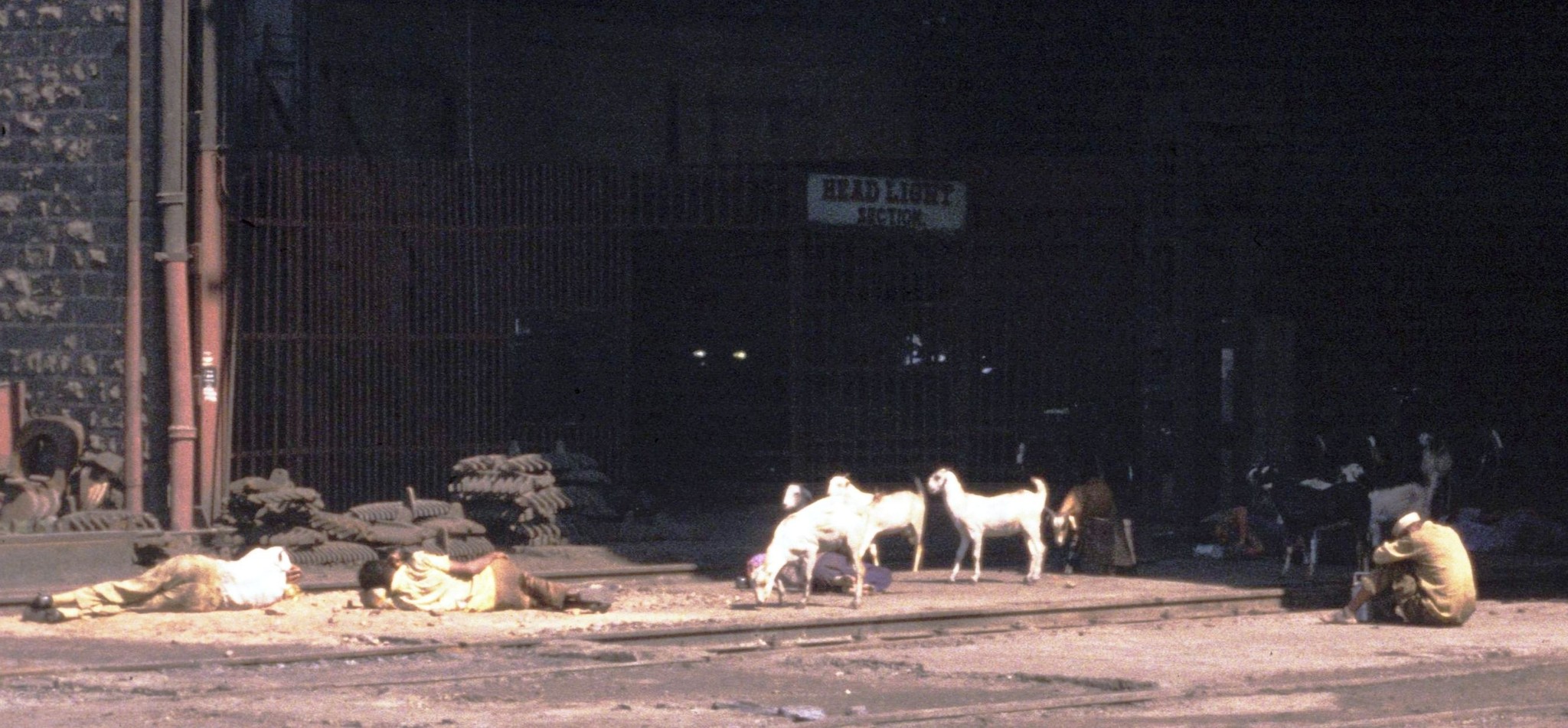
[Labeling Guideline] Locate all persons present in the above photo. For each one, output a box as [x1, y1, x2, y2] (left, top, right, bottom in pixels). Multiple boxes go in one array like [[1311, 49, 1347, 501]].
[[33, 546, 302, 624], [359, 550, 614, 613], [1318, 510, 1477, 626]]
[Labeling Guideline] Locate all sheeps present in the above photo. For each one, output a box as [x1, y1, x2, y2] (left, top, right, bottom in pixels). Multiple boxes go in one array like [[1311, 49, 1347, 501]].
[[1243, 438, 1458, 579], [925, 465, 1047, 584], [1048, 476, 1117, 572], [745, 471, 927, 608]]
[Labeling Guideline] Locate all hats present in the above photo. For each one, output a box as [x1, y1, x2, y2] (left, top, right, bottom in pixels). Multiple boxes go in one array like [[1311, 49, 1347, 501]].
[[1392, 512, 1420, 538]]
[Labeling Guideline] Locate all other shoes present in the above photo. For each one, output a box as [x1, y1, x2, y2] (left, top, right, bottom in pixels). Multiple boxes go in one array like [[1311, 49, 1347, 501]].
[[577, 587, 615, 614], [22, 595, 66, 623]]
